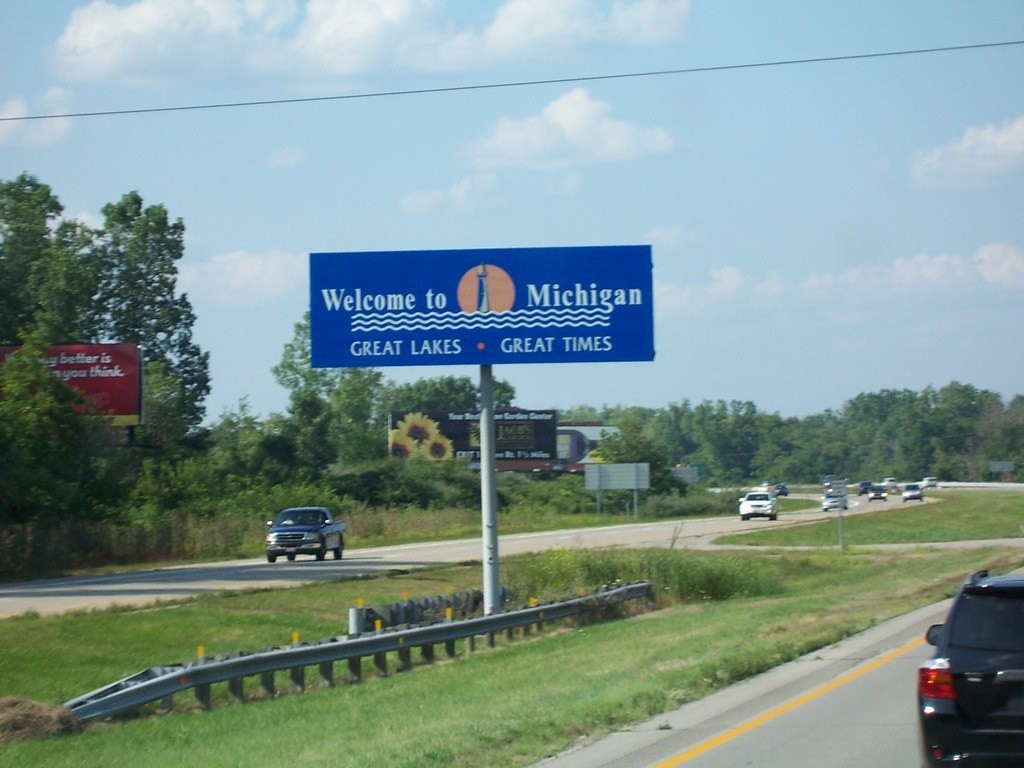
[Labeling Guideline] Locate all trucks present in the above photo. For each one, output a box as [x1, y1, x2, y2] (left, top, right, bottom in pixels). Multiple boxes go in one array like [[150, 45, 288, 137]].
[[822, 473, 845, 490]]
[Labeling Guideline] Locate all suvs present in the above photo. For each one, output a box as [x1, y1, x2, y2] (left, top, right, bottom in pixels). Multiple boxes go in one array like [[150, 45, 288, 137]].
[[916, 569, 1024, 768]]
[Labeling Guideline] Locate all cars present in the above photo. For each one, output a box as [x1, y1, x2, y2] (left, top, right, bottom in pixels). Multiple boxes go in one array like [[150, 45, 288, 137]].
[[899, 484, 923, 502], [857, 481, 872, 496], [821, 493, 847, 511], [884, 478, 896, 487], [867, 483, 888, 501], [762, 481, 772, 486], [773, 485, 788, 496], [921, 477, 937, 487]]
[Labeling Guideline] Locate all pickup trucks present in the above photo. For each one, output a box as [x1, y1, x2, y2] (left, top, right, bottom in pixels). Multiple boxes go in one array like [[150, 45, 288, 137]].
[[739, 492, 777, 521], [265, 508, 345, 564]]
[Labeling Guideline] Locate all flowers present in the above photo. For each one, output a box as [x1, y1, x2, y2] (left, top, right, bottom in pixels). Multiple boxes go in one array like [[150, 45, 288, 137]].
[[390, 412, 454, 462]]
